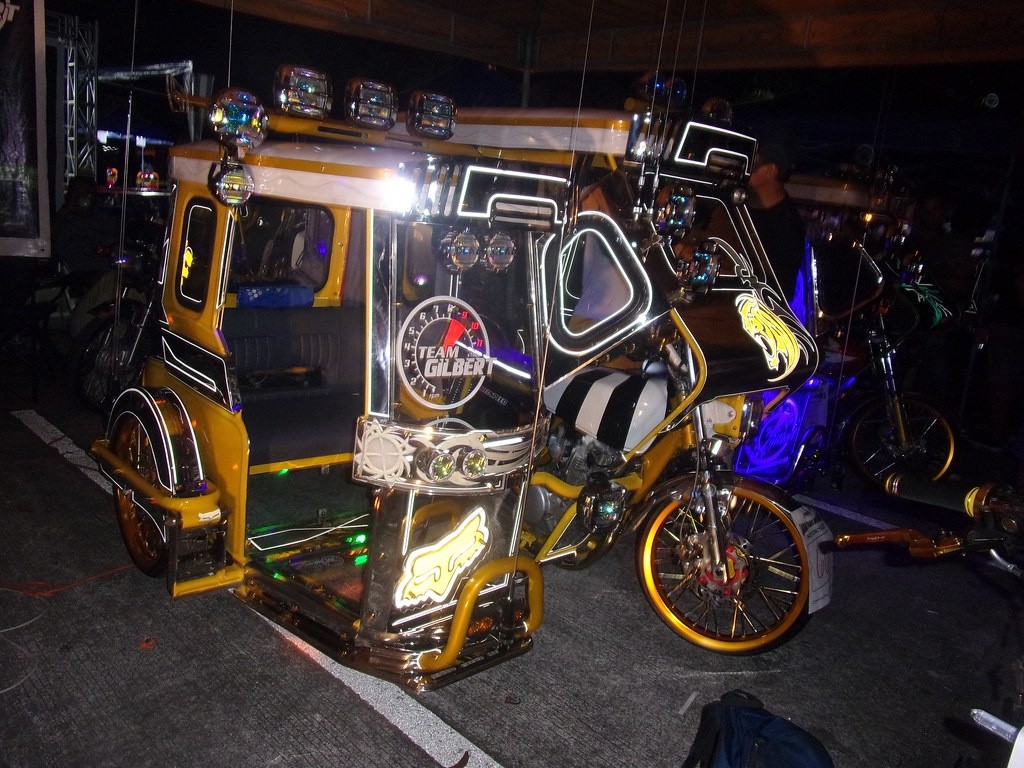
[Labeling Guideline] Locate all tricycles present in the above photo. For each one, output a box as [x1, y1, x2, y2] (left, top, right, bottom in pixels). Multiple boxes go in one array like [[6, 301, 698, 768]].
[[31, 68, 1024, 696]]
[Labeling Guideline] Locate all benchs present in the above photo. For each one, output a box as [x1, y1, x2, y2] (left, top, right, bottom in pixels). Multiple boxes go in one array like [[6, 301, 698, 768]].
[[221, 305, 366, 407]]
